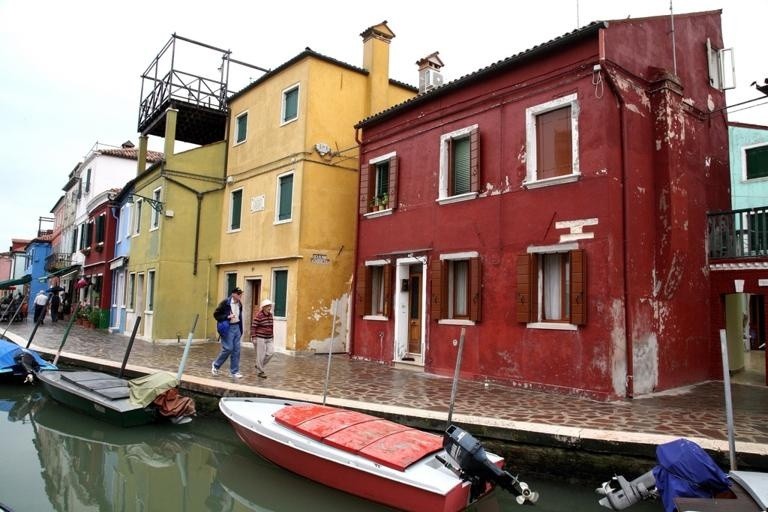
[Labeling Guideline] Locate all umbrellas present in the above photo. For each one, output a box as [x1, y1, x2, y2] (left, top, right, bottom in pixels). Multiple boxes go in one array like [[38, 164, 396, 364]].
[[46, 285, 65, 292]]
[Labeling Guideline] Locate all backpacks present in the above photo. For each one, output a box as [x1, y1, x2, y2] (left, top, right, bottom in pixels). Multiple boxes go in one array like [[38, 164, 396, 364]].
[[216, 302, 230, 336]]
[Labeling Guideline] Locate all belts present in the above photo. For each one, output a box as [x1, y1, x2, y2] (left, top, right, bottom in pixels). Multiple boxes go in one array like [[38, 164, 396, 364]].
[[230, 322, 240, 325]]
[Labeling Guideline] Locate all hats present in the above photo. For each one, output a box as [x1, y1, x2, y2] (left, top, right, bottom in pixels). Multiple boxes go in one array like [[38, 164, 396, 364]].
[[232, 287, 243, 293], [259, 300, 274, 311]]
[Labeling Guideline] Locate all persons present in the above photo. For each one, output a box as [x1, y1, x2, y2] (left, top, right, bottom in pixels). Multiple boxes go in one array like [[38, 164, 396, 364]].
[[33, 290, 48, 324], [250, 300, 275, 379], [0, 290, 30, 322], [36, 293, 47, 321], [61, 293, 71, 321], [49, 291, 61, 321], [210, 287, 244, 379], [206, 475, 236, 511]]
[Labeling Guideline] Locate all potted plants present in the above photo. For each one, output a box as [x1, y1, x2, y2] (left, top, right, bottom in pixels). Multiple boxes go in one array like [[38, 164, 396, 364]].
[[370, 192, 389, 212], [75, 306, 100, 330]]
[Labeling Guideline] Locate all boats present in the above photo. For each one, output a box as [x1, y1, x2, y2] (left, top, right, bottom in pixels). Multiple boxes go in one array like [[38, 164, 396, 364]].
[[29, 358, 198, 429], [0, 338, 62, 389], [591, 437, 768, 512], [215, 395, 545, 512]]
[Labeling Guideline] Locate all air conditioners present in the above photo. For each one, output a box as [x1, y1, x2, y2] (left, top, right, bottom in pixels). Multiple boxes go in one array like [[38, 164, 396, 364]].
[[424, 69, 445, 92]]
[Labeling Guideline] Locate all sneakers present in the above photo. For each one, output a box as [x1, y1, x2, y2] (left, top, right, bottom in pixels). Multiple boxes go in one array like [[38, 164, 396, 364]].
[[230, 372, 243, 379], [257, 372, 267, 378], [211, 361, 219, 377], [255, 365, 264, 373]]
